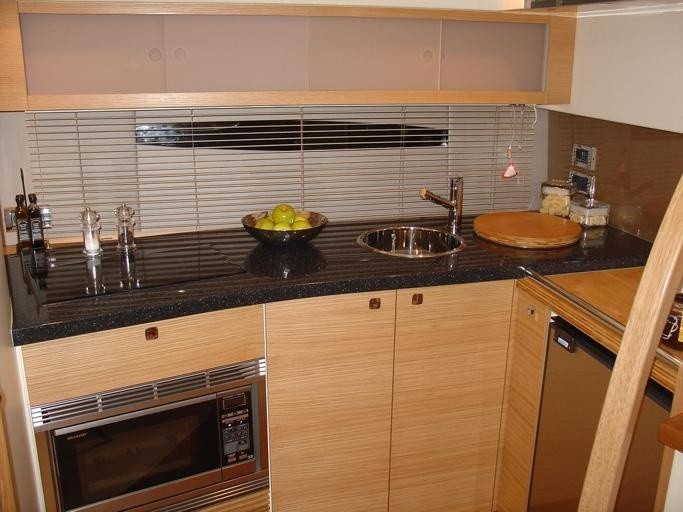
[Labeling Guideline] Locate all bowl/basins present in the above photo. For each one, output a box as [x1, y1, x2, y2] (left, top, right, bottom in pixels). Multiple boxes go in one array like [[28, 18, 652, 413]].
[[242, 242, 329, 281], [238, 208, 330, 248]]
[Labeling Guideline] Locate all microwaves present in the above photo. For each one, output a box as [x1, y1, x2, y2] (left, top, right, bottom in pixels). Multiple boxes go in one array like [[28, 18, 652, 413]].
[[28, 356, 271, 512]]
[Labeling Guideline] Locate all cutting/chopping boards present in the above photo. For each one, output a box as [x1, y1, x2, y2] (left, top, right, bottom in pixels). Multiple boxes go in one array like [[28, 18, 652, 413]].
[[472, 207, 583, 250]]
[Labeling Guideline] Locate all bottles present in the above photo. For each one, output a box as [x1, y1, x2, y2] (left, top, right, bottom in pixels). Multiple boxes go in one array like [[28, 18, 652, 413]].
[[14, 194, 34, 254], [78, 208, 105, 258], [26, 193, 46, 252], [83, 252, 109, 295], [21, 250, 49, 296], [113, 202, 138, 251], [118, 250, 141, 292]]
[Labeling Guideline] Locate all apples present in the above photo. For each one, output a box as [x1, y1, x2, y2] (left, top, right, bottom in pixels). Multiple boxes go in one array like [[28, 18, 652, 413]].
[[255, 204, 312, 232]]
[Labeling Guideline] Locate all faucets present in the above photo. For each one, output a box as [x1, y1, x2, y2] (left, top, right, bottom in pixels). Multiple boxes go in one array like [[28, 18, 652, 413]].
[[418, 177, 464, 227]]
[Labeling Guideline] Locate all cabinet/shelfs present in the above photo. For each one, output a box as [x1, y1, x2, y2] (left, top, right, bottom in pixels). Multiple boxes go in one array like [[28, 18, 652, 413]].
[[264, 277, 514, 512], [21, 302, 265, 408], [0, 1, 578, 112]]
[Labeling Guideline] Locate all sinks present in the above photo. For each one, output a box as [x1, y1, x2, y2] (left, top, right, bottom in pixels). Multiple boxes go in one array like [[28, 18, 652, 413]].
[[356, 225, 466, 259]]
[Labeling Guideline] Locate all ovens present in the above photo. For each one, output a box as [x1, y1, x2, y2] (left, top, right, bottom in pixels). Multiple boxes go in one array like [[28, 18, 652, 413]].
[[525, 318, 671, 511]]
